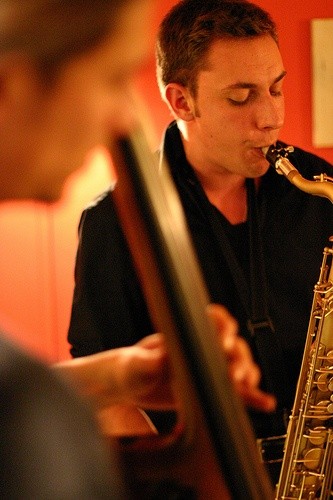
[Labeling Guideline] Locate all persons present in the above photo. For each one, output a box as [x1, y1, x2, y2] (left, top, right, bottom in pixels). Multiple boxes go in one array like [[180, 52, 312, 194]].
[[69, 0, 333, 499], [0, 0, 276, 500]]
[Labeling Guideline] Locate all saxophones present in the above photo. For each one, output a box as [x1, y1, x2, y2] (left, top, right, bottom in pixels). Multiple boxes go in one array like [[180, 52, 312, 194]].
[[247, 142, 333, 500]]
